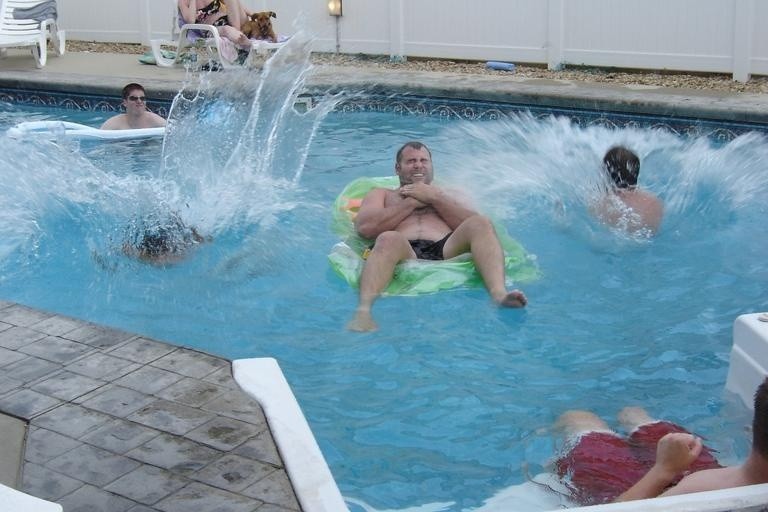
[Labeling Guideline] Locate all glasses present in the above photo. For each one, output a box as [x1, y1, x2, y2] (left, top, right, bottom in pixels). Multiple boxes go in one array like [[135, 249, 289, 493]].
[[129, 96, 145, 101]]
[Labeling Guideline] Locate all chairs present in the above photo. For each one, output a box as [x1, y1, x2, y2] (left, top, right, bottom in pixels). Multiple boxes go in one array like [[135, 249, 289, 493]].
[[149, 1, 297, 72], [0, 1, 68, 70]]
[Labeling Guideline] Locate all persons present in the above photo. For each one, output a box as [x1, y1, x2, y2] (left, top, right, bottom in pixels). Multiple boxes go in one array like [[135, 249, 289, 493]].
[[99, 82, 166, 131], [112, 210, 206, 266], [588, 146, 663, 235], [343, 142, 528, 331], [178, 0, 253, 46], [537, 372, 767, 506]]
